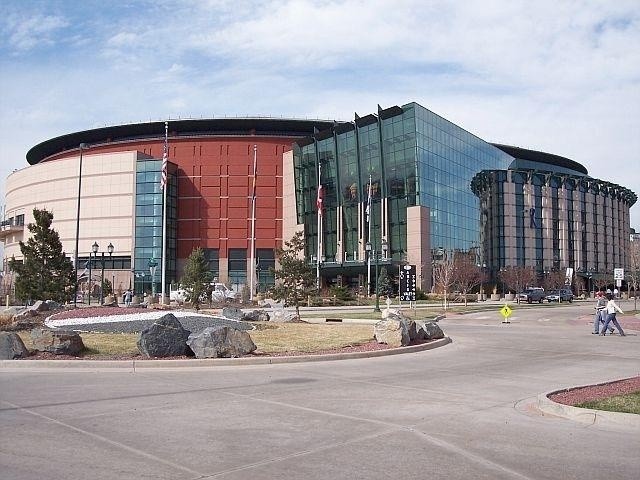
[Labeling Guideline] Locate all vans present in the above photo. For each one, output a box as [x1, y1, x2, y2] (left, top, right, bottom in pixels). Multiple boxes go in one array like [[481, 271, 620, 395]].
[[177, 283, 241, 303]]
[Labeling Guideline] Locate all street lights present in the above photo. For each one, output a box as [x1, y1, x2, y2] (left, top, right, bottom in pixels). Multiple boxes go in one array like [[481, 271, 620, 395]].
[[74, 142, 90, 303], [312, 254, 326, 297], [477, 260, 487, 301], [365, 240, 388, 312], [586, 272, 592, 297], [92, 241, 114, 305]]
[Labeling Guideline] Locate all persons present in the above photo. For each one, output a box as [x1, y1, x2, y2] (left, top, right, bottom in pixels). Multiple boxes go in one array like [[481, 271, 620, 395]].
[[591, 291, 615, 334], [123, 289, 135, 308], [598, 293, 625, 336]]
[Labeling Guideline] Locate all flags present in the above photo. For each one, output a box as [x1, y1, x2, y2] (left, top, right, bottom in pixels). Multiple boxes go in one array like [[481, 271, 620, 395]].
[[158, 131, 169, 192], [316, 167, 325, 218], [364, 184, 372, 224], [252, 151, 258, 202]]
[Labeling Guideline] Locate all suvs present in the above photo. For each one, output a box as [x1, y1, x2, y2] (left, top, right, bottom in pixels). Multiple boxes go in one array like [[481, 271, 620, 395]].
[[516, 288, 574, 303]]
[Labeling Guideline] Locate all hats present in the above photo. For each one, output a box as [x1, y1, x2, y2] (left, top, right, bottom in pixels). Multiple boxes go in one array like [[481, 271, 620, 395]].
[[596, 291, 601, 296]]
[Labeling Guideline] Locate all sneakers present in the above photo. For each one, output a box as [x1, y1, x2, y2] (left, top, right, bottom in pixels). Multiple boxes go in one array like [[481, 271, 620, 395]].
[[592, 331, 599, 334], [610, 329, 614, 334]]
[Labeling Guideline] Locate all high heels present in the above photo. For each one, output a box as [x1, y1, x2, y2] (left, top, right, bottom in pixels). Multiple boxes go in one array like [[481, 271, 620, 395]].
[[599, 331, 605, 336]]
